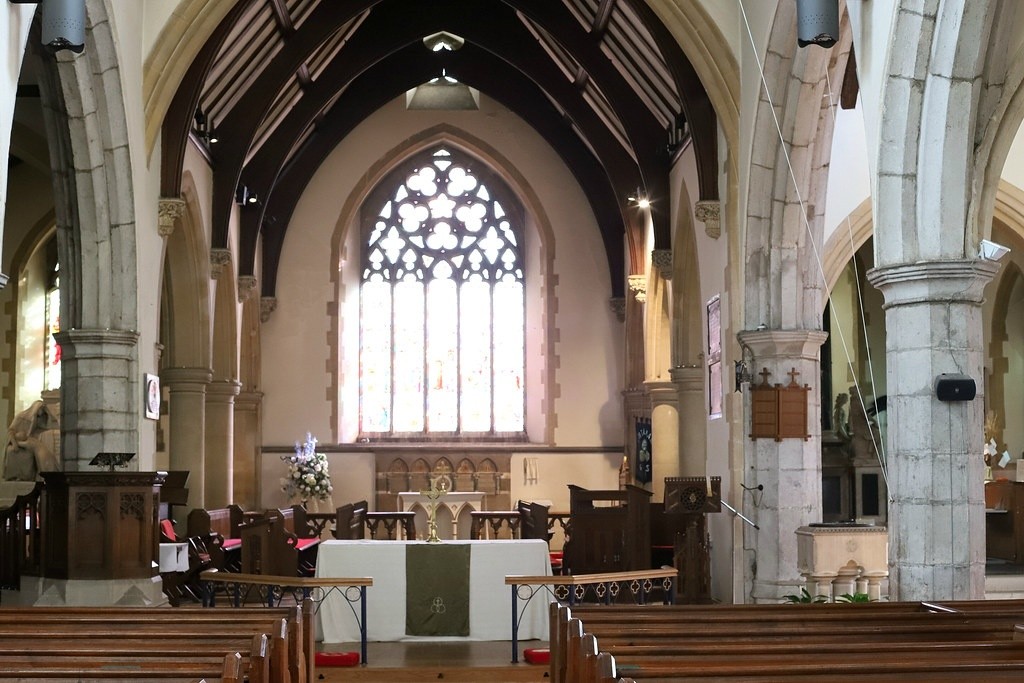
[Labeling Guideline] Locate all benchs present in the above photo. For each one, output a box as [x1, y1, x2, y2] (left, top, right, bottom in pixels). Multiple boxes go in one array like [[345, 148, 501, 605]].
[[158, 500, 370, 581], [549, 599, 1024, 683], [0, 597, 315, 683]]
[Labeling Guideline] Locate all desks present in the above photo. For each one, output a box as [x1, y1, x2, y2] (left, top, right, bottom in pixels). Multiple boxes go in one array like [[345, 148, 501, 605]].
[[318, 539, 546, 642]]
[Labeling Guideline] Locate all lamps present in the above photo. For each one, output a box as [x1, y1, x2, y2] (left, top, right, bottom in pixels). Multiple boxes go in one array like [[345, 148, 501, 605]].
[[980, 240, 1012, 261]]
